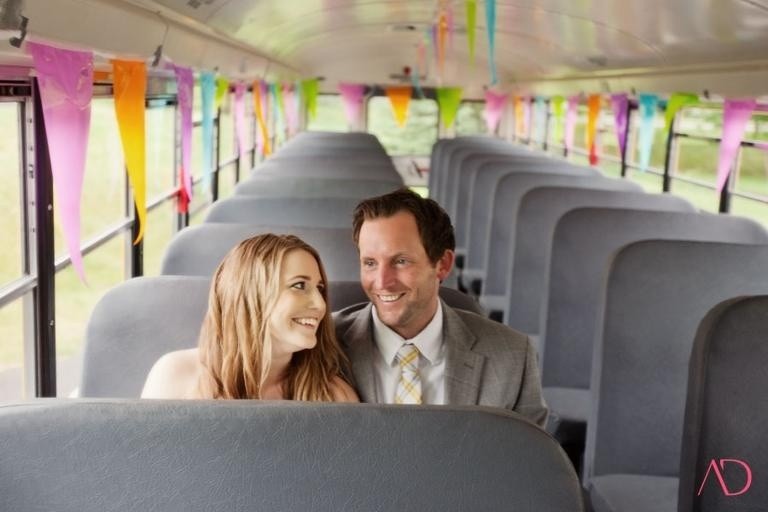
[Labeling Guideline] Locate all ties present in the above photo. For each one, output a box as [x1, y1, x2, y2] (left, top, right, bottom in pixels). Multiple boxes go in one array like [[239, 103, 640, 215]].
[[395, 343, 422, 404]]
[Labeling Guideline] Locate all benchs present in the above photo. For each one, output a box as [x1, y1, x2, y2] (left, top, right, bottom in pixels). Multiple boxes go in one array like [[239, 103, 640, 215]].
[[3, 129, 768, 512]]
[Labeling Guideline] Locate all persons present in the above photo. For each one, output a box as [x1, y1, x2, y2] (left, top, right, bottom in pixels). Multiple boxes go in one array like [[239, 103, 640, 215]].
[[139, 233, 358, 402], [324, 188, 550, 430]]
